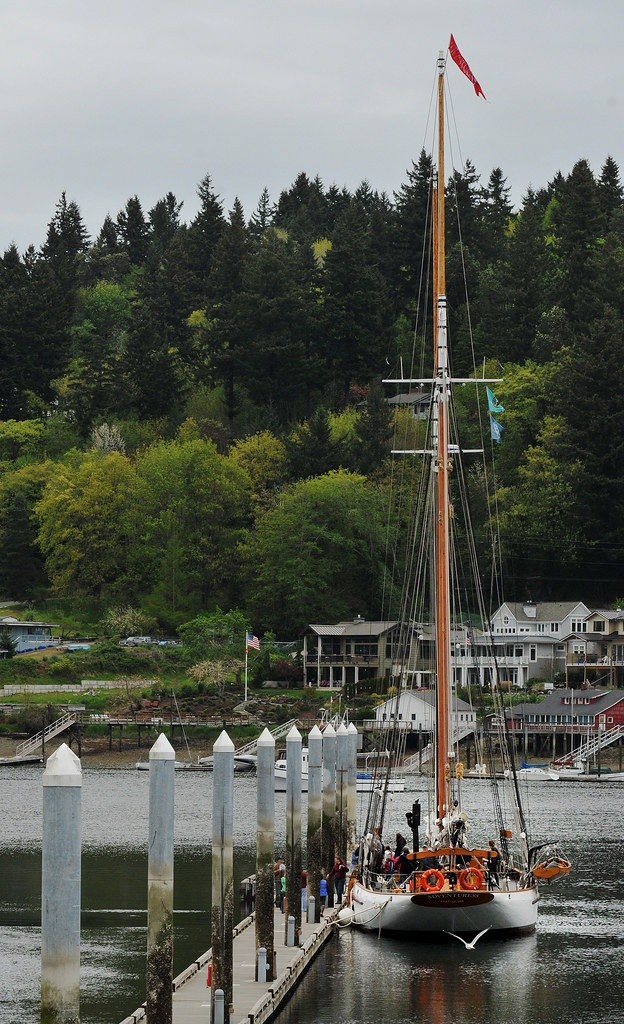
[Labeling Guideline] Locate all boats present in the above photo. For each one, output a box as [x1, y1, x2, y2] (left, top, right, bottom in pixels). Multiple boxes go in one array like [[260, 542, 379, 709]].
[[504, 768, 560, 782], [274, 695, 405, 793], [532, 857, 571, 885], [548, 760, 585, 776]]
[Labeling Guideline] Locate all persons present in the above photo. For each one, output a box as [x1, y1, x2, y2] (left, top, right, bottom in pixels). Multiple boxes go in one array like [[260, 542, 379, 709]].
[[487, 840, 502, 886], [351, 833, 439, 892], [319, 874, 328, 917], [299, 867, 308, 912], [274, 856, 288, 914], [326, 856, 349, 904]]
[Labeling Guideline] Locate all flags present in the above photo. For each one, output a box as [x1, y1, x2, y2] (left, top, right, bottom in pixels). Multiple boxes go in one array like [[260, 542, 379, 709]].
[[465, 629, 474, 646], [245, 632, 261, 651]]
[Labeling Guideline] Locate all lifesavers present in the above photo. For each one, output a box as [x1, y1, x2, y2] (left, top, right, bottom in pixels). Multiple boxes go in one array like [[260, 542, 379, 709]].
[[421, 869, 444, 892], [459, 868, 483, 891]]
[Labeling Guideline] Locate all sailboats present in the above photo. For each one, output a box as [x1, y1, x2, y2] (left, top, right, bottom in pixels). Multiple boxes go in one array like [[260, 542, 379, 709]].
[[136, 688, 193, 770], [348, 49, 540, 948]]
